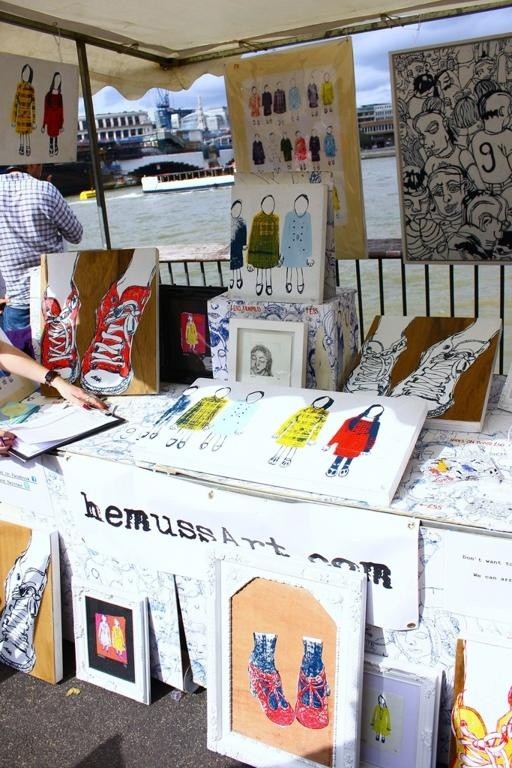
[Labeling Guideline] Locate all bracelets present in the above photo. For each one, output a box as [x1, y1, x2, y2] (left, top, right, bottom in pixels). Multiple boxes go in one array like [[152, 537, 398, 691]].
[[45, 371, 61, 387]]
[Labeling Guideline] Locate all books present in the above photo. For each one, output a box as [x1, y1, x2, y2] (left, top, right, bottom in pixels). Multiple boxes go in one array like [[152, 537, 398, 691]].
[[2, 405, 127, 465]]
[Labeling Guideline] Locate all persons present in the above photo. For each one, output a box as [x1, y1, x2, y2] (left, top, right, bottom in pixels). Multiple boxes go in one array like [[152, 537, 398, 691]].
[[250, 343, 273, 378], [0, 339, 111, 458]]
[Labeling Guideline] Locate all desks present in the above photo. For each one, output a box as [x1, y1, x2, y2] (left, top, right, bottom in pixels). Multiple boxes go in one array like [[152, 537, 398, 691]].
[[1, 386, 512, 694]]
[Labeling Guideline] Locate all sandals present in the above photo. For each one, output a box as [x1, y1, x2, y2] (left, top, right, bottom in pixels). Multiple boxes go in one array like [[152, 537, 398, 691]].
[[198, 442, 222, 454], [297, 282, 304, 294], [265, 280, 272, 296], [167, 437, 185, 449], [229, 278, 235, 290], [141, 431, 159, 440], [256, 282, 263, 296], [326, 467, 348, 479], [236, 278, 243, 289], [285, 282, 294, 293]]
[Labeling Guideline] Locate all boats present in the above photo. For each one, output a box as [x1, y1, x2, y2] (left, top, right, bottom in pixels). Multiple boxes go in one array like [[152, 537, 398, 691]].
[[138, 166, 237, 193], [78, 186, 95, 200], [86, 167, 137, 189]]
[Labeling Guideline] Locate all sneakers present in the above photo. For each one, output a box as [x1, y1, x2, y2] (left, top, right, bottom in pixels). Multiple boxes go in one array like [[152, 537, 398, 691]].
[[79, 279, 152, 396], [387, 330, 491, 420], [269, 455, 291, 470], [339, 335, 407, 396], [40, 278, 80, 384], [0, 554, 48, 672], [450, 688, 512, 768]]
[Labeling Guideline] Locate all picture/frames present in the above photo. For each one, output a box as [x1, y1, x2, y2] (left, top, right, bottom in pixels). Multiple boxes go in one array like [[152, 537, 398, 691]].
[[359, 654, 443, 768], [70, 577, 150, 705], [388, 32, 512, 264], [229, 319, 307, 388], [207, 542, 367, 768]]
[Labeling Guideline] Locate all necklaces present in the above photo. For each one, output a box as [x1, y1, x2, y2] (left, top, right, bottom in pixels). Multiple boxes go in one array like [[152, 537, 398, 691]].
[[0, 164, 85, 336]]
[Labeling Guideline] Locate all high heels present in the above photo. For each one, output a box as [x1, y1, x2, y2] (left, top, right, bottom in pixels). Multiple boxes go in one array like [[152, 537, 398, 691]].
[[295, 666, 330, 729], [248, 661, 295, 726]]
